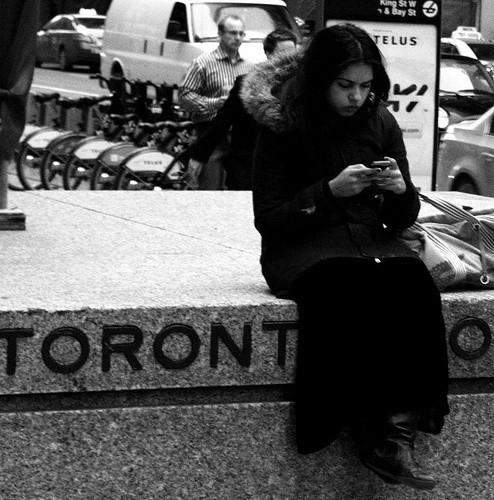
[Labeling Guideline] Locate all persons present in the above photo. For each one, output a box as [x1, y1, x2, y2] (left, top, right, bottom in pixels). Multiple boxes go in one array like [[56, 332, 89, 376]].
[[188, 28, 299, 190], [179, 14, 248, 190], [240, 24, 451, 489]]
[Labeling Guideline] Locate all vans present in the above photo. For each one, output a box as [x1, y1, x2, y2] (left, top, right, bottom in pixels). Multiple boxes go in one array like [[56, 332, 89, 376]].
[[99, 0, 309, 100]]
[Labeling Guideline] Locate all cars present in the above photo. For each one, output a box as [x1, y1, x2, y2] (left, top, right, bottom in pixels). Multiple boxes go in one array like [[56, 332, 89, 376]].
[[33, 13, 107, 73], [436, 26, 494, 200]]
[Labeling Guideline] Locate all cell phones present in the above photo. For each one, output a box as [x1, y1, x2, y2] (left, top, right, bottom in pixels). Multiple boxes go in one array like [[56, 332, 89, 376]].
[[369, 161, 391, 169]]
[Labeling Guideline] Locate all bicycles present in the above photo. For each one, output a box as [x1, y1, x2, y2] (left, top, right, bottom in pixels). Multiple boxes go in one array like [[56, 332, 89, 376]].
[[4, 72, 200, 191]]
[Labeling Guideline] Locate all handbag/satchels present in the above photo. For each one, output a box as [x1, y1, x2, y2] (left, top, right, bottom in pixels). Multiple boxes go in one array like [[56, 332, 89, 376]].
[[399, 191, 494, 292]]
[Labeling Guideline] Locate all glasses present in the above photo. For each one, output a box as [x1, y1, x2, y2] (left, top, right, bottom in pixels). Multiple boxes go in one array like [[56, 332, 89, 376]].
[[223, 30, 246, 38]]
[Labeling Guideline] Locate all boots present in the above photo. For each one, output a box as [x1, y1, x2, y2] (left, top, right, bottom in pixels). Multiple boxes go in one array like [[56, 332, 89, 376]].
[[361, 405, 437, 491]]
